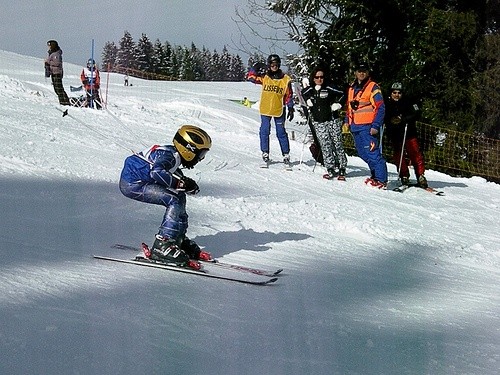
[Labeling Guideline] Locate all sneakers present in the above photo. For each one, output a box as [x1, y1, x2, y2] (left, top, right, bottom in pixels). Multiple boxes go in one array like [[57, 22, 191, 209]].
[[401, 177, 410, 187], [149, 232, 189, 264], [417, 175, 427, 186], [364, 178, 387, 190], [177, 234, 201, 255]]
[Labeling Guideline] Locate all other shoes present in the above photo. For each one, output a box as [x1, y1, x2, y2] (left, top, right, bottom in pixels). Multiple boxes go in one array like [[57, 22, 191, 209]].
[[262, 153, 269, 162], [323, 171, 336, 180], [336, 176, 345, 181], [283, 154, 290, 165]]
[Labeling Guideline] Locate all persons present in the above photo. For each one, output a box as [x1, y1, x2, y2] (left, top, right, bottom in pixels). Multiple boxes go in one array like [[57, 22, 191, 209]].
[[118, 124, 212, 266], [384, 81, 430, 189], [124, 74, 129, 86], [80, 58, 103, 110], [300, 65, 349, 177], [44, 38, 71, 106], [246, 53, 296, 165], [343, 63, 389, 190], [243, 96, 251, 108]]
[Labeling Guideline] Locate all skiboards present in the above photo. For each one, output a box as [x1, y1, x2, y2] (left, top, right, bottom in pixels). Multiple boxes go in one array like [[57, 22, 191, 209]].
[[393, 184, 444, 195], [92, 242, 282, 285], [323, 174, 346, 181], [260, 157, 292, 171]]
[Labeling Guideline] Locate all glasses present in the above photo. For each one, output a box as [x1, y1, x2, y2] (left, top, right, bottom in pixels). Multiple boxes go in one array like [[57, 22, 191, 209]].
[[393, 91, 401, 94], [358, 70, 367, 73], [314, 76, 323, 79]]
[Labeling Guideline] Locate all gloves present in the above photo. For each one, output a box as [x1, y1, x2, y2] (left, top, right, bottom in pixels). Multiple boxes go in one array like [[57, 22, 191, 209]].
[[177, 176, 200, 195], [253, 62, 268, 72], [287, 107, 295, 121], [307, 99, 313, 106], [331, 103, 341, 111]]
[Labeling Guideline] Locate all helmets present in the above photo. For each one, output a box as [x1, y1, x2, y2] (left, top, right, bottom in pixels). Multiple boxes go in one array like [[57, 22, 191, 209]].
[[87, 59, 96, 64], [173, 125, 212, 162], [47, 40, 58, 49], [267, 54, 281, 68]]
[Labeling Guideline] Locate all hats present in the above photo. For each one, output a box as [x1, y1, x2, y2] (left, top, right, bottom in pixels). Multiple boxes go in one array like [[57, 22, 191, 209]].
[[391, 82, 402, 89], [355, 63, 370, 70]]
